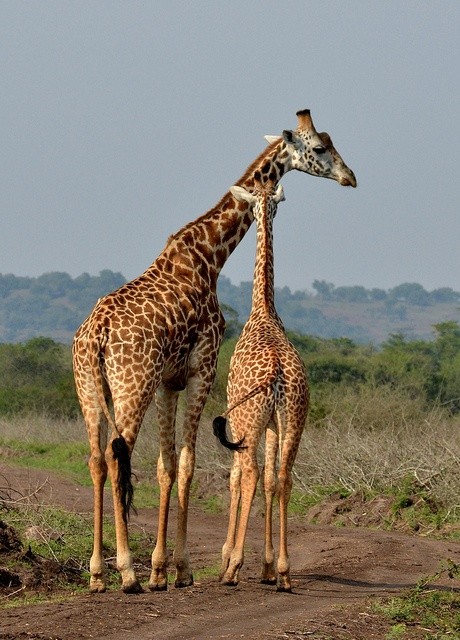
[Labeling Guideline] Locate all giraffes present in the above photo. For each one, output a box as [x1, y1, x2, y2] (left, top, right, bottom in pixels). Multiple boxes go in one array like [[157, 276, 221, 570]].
[[211, 170, 311, 592], [71, 109, 357, 594]]
